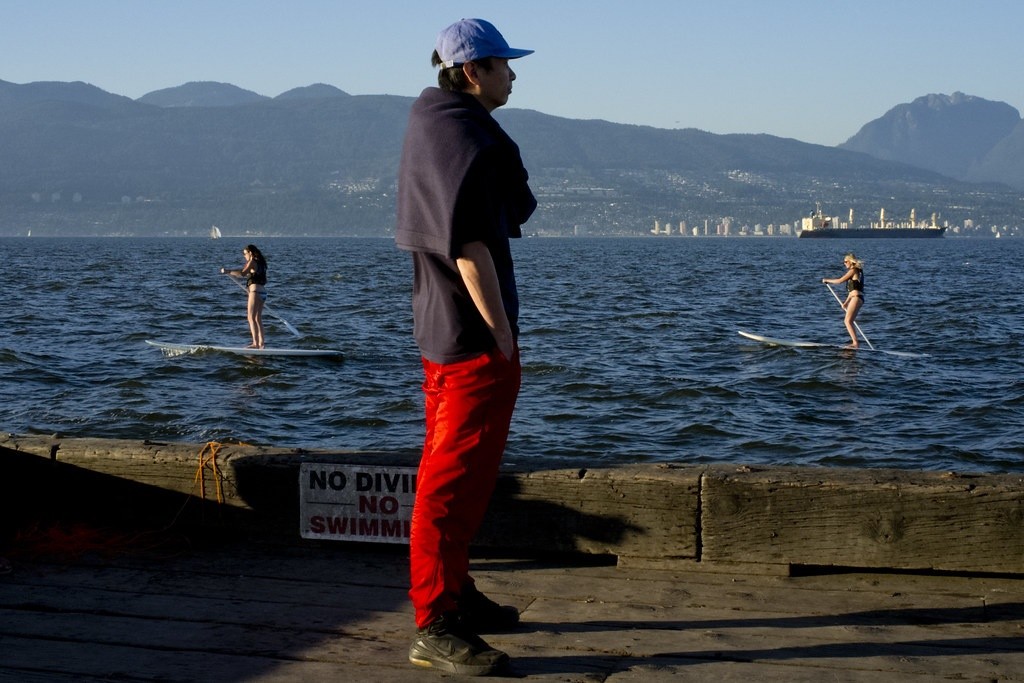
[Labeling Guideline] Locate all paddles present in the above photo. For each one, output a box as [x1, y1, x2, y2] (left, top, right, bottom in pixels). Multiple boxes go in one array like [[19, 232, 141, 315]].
[[824, 281, 875, 350], [225, 273, 300, 336]]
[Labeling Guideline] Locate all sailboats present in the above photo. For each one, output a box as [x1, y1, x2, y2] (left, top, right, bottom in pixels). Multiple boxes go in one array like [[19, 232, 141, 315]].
[[209, 226, 222, 239]]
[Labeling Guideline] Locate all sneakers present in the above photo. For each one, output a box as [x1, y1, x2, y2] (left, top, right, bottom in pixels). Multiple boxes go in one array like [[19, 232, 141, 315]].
[[438, 583, 519, 633], [407, 610, 511, 676]]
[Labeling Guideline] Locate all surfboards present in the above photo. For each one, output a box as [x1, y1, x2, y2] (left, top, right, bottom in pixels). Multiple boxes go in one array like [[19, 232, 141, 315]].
[[144, 339, 348, 358], [737, 329, 932, 359]]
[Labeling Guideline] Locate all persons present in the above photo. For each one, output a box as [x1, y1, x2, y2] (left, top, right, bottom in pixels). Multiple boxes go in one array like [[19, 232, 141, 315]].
[[396, 17, 537, 678], [823, 254, 866, 347], [221, 245, 268, 349]]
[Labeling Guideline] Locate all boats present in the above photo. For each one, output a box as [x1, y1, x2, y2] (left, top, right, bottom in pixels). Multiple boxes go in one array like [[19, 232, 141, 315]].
[[799, 226, 947, 238]]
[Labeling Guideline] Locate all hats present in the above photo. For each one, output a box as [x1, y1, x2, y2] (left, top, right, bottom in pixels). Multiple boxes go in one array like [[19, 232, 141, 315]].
[[435, 17, 535, 70]]
